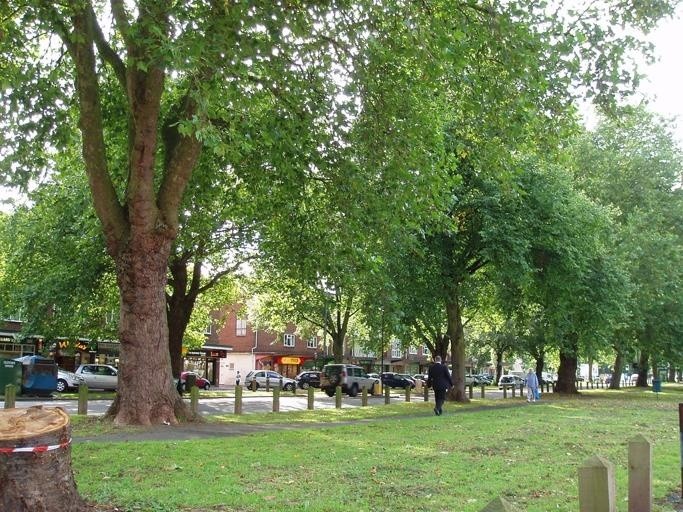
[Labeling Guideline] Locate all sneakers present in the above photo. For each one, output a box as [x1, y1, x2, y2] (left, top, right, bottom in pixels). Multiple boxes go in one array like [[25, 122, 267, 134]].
[[434, 407, 440, 416], [526, 399, 535, 403]]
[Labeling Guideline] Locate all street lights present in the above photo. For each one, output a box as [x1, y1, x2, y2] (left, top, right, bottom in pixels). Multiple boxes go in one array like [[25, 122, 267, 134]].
[[378, 306, 384, 394]]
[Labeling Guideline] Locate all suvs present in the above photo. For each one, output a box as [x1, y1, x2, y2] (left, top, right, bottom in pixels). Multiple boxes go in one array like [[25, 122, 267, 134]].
[[245, 370, 297, 391], [75, 364, 119, 388], [319, 364, 374, 398], [295, 371, 320, 390]]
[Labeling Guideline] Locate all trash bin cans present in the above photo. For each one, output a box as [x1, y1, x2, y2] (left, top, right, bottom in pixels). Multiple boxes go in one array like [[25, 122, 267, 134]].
[[652, 380, 661, 392], [185, 373, 196, 393]]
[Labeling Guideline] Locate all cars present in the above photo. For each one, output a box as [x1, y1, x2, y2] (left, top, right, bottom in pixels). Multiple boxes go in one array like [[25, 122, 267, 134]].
[[498, 375, 524, 390], [605, 377, 612, 384], [181, 372, 210, 391], [631, 374, 639, 381], [56, 369, 86, 392], [465, 373, 494, 386], [367, 372, 428, 389]]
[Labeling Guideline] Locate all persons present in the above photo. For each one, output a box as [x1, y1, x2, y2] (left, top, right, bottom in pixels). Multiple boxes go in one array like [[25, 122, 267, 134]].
[[425, 356, 454, 416], [234, 370, 241, 385], [523, 367, 538, 402]]
